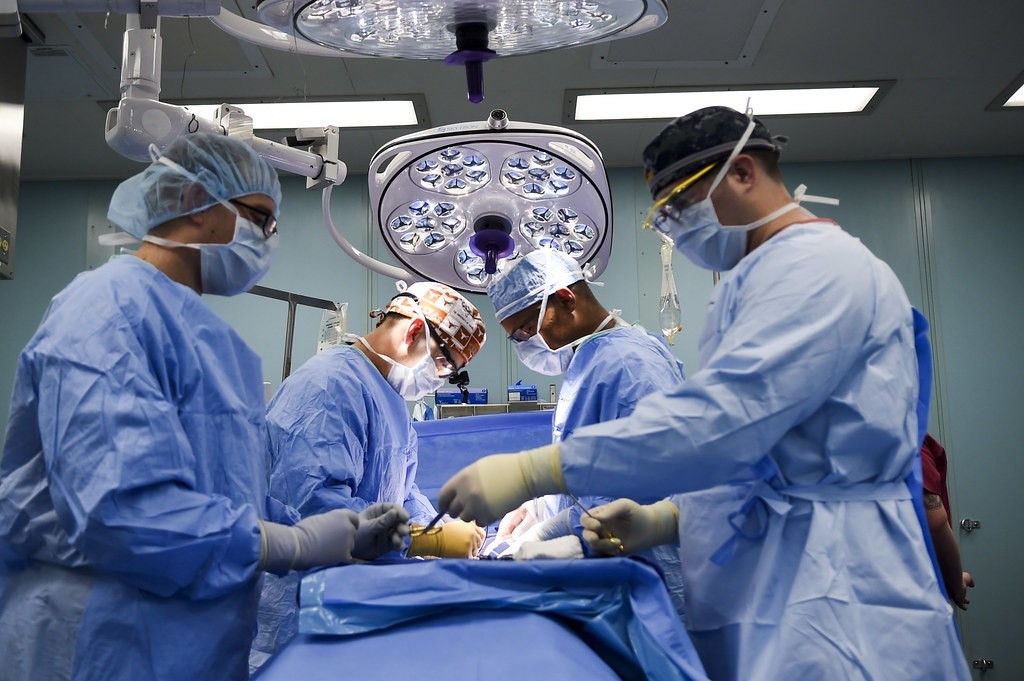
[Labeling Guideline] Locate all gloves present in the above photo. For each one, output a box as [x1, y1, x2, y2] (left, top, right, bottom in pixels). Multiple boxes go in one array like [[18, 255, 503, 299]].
[[409, 522, 486, 558], [350, 502, 409, 560], [258, 507, 359, 577]]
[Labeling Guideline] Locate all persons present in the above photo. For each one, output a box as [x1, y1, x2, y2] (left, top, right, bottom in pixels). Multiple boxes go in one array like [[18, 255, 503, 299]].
[[0, 134, 410, 681], [246, 281, 489, 676], [488, 246, 692, 619], [919, 424, 974, 609], [437, 108, 973, 681]]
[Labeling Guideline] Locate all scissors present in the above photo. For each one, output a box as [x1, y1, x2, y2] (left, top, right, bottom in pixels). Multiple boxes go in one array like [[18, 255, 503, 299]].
[[408, 508, 447, 538]]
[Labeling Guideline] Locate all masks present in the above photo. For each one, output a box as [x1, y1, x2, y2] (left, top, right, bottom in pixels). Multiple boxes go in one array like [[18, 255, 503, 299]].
[[388, 337, 444, 402], [660, 164, 767, 272], [189, 203, 273, 296], [512, 308, 585, 377]]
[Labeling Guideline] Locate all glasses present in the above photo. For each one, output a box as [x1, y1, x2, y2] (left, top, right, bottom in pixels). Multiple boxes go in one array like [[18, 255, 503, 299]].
[[654, 159, 729, 234], [507, 293, 553, 344], [233, 200, 277, 239]]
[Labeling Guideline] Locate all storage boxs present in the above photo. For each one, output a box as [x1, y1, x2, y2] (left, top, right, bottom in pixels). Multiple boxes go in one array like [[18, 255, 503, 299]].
[[507, 385, 537, 401], [435, 388, 488, 405]]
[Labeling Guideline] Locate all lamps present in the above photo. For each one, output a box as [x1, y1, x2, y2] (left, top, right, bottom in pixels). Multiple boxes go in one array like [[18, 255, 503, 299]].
[[368, 108, 613, 295], [251, 0, 669, 104]]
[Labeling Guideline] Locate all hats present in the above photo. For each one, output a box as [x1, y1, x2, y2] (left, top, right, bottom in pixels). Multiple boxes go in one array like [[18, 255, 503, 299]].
[[386, 280, 486, 366], [643, 106, 781, 199], [488, 248, 585, 324]]
